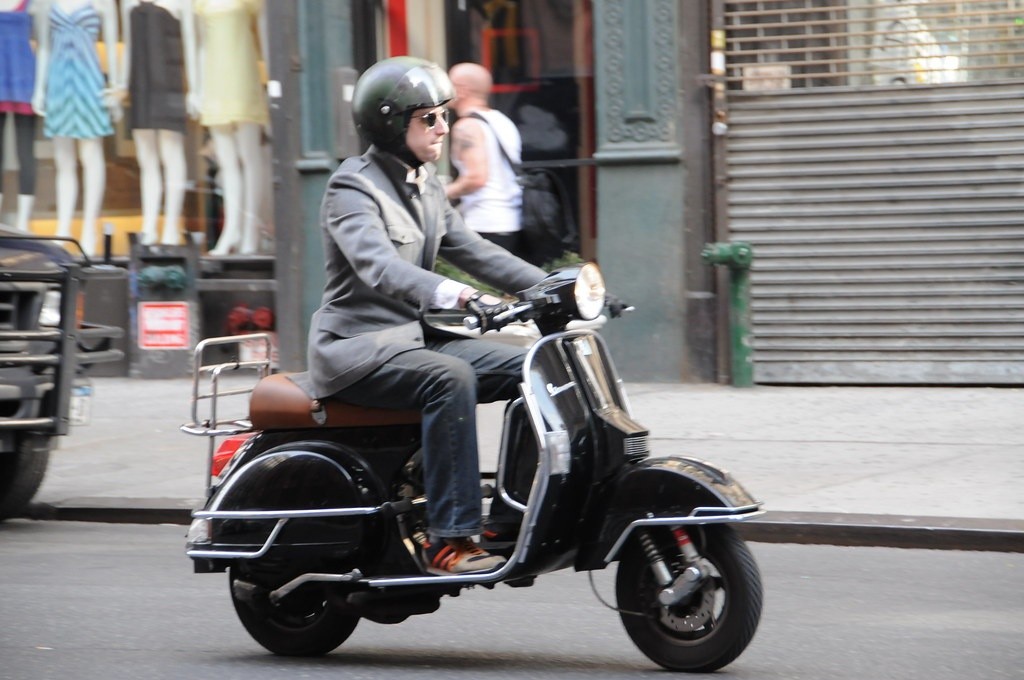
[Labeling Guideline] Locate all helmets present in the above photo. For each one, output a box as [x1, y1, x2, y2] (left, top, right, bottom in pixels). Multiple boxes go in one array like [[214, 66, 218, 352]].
[[352, 56, 454, 145]]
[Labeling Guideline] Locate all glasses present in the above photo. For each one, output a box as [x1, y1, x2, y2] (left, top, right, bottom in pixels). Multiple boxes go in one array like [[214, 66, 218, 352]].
[[409, 108, 449, 127]]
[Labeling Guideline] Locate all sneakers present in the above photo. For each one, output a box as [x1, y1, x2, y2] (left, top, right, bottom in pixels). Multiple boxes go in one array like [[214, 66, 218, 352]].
[[481, 517, 513, 542], [422, 538, 506, 575]]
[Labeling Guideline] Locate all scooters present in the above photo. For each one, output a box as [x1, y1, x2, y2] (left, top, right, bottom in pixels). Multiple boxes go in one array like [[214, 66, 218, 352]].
[[178, 256, 764, 673]]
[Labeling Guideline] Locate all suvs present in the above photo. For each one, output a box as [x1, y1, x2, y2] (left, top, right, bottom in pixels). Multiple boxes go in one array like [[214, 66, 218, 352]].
[[1, 226, 130, 520]]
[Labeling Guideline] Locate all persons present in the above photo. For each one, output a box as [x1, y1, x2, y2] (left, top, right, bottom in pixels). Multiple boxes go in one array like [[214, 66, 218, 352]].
[[29, 0, 123, 261], [439, 63, 526, 260], [119, 1, 197, 246], [306, 56, 530, 572], [0, 0, 35, 234], [194, 1, 268, 253]]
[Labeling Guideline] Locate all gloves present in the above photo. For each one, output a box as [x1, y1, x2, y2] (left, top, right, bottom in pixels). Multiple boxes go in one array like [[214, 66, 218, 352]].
[[466, 292, 514, 333], [604, 291, 630, 321]]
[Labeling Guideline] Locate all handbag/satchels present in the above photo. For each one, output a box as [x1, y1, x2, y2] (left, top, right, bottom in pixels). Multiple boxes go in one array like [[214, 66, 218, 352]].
[[523, 182, 564, 256]]
